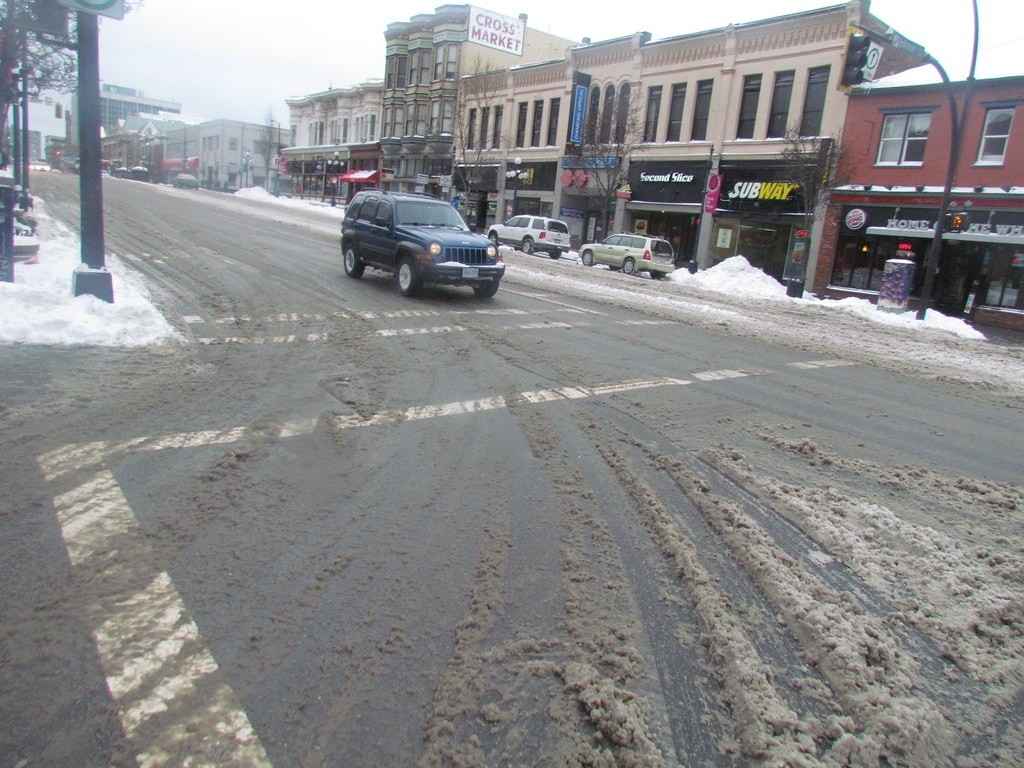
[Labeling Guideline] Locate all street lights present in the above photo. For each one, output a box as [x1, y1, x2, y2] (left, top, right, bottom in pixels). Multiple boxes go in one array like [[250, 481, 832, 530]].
[[271, 120, 280, 196], [242, 152, 252, 187], [327, 152, 345, 206], [506, 157, 528, 217]]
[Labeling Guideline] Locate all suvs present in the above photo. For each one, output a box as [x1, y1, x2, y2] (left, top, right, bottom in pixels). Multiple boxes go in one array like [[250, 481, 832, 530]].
[[340, 188, 506, 297], [578, 232, 675, 279], [487, 215, 570, 259]]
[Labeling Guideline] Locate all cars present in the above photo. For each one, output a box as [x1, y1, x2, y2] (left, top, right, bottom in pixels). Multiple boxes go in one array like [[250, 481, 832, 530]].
[[30, 154, 149, 181], [172, 173, 199, 189]]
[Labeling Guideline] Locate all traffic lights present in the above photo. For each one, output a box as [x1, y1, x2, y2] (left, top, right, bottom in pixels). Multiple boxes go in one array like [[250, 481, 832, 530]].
[[944, 214, 961, 231], [837, 27, 872, 94], [144, 136, 150, 148]]
